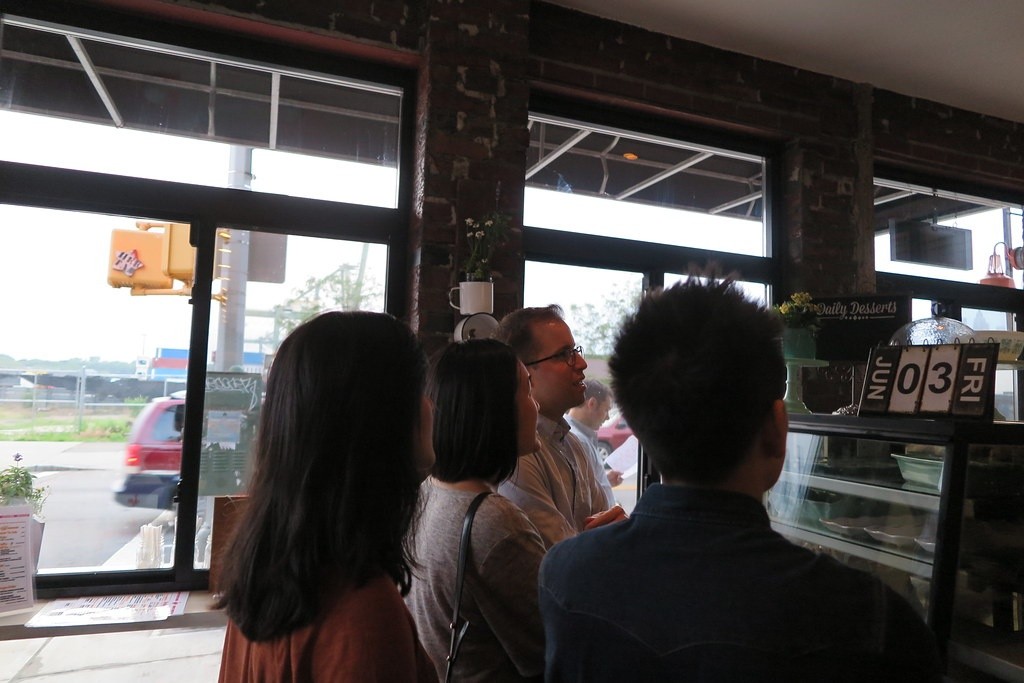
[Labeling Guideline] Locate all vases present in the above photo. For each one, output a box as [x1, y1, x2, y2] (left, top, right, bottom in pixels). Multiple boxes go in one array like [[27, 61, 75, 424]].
[[781, 312, 817, 328], [465, 272, 492, 282]]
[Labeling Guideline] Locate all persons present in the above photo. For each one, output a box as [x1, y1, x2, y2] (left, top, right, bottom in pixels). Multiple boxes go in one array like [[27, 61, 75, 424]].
[[537, 262, 943, 683], [208, 312, 439, 683], [563, 380, 623, 509], [398, 338, 547, 683], [492, 304, 631, 550]]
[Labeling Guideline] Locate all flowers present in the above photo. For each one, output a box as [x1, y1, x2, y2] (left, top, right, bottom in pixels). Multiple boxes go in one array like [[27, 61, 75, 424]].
[[779, 293, 818, 315], [463, 212, 501, 271]]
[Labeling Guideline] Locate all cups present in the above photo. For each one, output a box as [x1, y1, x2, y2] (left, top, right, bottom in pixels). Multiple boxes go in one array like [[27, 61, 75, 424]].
[[454, 311, 501, 345], [448, 281, 495, 316]]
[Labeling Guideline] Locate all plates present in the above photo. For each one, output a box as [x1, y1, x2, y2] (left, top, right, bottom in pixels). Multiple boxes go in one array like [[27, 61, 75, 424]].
[[819, 515, 935, 554]]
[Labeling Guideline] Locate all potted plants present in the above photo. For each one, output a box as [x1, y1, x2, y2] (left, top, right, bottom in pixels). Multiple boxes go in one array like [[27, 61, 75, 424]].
[[0, 452, 45, 516]]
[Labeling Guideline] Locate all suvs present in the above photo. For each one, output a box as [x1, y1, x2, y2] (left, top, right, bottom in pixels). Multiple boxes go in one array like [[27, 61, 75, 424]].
[[114, 386, 269, 508]]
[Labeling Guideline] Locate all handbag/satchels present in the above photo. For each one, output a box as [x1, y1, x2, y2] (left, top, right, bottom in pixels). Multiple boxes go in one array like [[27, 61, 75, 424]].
[[445, 607, 543, 682]]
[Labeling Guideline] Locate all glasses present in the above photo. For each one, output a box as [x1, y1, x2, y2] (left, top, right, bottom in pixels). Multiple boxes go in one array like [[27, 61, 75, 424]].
[[525, 345, 583, 365]]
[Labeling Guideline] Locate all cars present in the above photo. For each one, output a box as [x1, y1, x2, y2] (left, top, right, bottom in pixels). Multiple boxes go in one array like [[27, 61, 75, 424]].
[[596, 406, 635, 467]]
